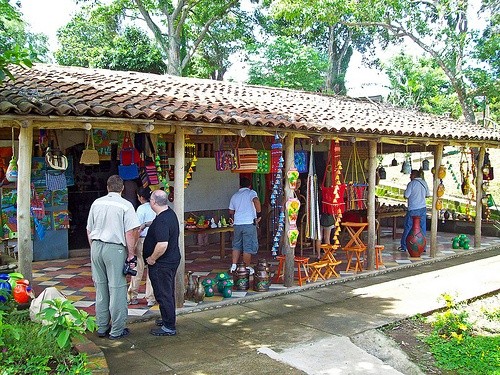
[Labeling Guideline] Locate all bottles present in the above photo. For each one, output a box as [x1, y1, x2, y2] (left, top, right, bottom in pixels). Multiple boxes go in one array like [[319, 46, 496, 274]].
[[452, 234, 471, 250], [202, 258, 272, 298], [406, 215, 426, 257], [0, 272, 35, 306], [5, 156, 19, 183]]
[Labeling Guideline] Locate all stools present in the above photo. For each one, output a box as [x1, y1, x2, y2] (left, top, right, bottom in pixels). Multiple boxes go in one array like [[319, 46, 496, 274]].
[[275, 243, 343, 287], [341, 222, 384, 275]]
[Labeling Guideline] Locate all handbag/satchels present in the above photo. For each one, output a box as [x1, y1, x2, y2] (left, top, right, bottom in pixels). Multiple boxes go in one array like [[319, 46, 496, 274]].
[[5, 156, 18, 182], [213, 135, 282, 174], [79, 148, 100, 165], [116, 148, 160, 188], [294, 150, 309, 173], [46, 147, 69, 170], [482, 159, 495, 181], [321, 180, 368, 216]]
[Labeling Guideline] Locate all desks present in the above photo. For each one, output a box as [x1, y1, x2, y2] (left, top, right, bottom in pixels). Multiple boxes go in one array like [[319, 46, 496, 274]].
[[184, 227, 234, 259], [360, 210, 408, 245]]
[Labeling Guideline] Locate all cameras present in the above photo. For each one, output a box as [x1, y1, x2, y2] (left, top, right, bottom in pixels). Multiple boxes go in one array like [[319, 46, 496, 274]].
[[123, 263, 137, 276]]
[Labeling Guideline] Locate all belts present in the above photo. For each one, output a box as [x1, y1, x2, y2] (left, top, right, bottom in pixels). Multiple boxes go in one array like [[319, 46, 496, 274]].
[[139, 236, 146, 239], [92, 239, 122, 245]]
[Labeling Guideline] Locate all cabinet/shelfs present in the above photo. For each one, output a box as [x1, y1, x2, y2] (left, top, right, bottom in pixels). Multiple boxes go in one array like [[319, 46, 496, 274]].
[[266, 206, 316, 256]]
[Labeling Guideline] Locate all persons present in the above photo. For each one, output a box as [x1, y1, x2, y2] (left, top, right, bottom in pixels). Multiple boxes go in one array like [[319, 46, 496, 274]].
[[229, 177, 261, 275], [398, 170, 429, 251], [86, 175, 180, 340]]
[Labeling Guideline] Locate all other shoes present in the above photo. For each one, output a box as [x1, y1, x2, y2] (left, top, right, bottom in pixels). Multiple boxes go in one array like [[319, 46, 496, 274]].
[[247, 267, 255, 275], [128, 299, 139, 305], [147, 301, 155, 307], [97, 326, 111, 338], [227, 267, 236, 274], [109, 328, 130, 340], [150, 326, 177, 336], [155, 319, 165, 327], [397, 247, 405, 252]]
